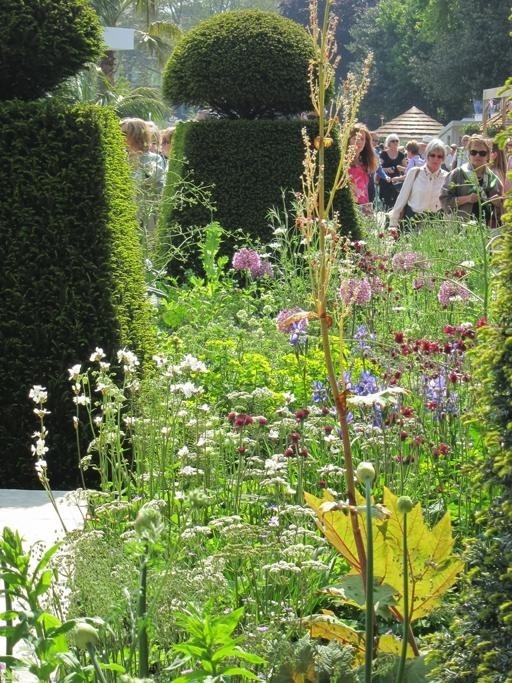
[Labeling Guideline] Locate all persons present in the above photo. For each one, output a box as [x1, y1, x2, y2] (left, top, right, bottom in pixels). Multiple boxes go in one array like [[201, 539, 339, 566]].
[[300, 108, 512, 240], [114, 109, 220, 251]]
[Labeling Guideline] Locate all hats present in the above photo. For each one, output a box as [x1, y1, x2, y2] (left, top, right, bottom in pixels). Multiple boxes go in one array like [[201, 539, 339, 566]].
[[385, 134, 399, 148]]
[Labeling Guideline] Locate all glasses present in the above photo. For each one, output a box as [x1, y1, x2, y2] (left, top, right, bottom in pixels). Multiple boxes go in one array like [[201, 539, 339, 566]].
[[428, 153, 443, 159], [392, 140, 398, 143], [469, 149, 488, 157]]
